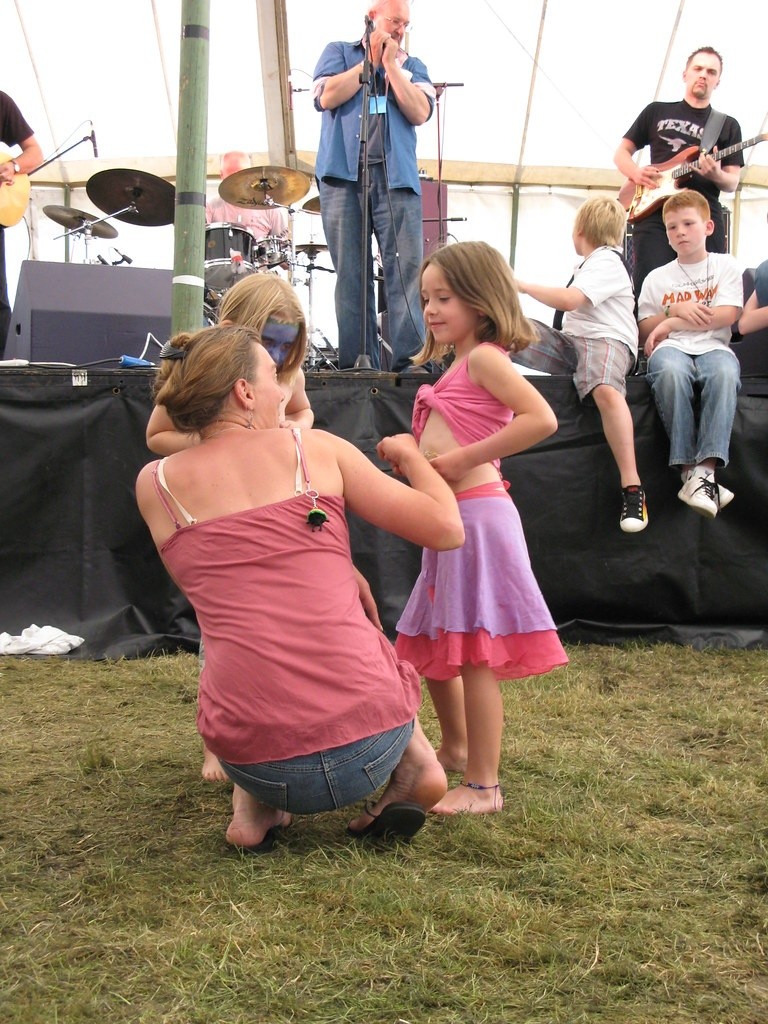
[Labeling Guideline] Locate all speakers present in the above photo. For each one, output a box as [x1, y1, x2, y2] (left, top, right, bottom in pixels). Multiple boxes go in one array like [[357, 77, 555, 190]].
[[3, 259, 173, 364]]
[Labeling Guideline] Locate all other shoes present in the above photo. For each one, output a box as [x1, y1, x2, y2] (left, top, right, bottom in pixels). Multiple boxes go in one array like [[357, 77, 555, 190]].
[[398, 363, 428, 374]]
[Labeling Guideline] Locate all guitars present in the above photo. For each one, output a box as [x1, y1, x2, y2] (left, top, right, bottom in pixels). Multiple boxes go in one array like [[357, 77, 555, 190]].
[[618, 133, 767, 225], [0, 151, 31, 228]]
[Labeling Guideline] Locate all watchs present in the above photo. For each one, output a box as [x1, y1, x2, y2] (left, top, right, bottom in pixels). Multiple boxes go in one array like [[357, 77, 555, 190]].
[[8, 160, 20, 174]]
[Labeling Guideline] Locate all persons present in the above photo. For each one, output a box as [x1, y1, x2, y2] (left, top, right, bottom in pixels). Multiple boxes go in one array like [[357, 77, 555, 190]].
[[739, 259, 768, 334], [147, 273, 313, 781], [613, 47, 744, 317], [0, 92, 43, 363], [394, 241, 568, 819], [512, 195, 648, 531], [134, 321, 466, 854], [636, 190, 744, 516], [312, 1, 436, 373], [206, 150, 291, 270]]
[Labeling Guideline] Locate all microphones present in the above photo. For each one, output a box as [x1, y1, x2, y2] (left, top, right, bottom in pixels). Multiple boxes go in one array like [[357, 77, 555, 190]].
[[90, 121, 97, 158], [365, 15, 376, 32], [288, 70, 293, 111]]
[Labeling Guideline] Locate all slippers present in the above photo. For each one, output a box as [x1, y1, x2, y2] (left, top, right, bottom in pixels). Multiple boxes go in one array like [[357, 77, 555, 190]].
[[345, 800, 427, 843], [232, 809, 293, 854]]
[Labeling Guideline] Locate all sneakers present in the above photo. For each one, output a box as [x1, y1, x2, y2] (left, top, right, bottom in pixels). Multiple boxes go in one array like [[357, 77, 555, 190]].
[[619, 481, 649, 533], [677, 473, 721, 519], [686, 469, 735, 509]]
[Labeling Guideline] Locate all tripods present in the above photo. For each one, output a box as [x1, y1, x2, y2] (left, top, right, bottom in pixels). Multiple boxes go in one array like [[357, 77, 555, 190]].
[[292, 256, 339, 372]]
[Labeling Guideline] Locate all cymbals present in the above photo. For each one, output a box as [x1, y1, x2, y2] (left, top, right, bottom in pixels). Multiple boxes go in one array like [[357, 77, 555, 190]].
[[218, 165, 312, 211], [44, 205, 118, 240], [86, 168, 176, 227], [301, 195, 320, 216], [295, 243, 328, 251]]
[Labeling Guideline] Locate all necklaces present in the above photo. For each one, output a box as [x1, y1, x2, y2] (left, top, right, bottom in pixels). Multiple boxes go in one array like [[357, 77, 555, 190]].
[[677, 252, 711, 308], [237, 214, 241, 222]]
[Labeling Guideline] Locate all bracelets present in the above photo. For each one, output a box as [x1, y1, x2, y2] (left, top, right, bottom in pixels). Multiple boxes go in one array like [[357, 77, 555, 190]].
[[665, 304, 671, 318]]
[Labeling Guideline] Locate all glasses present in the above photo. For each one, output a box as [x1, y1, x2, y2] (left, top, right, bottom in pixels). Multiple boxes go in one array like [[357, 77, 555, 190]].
[[374, 10, 412, 32]]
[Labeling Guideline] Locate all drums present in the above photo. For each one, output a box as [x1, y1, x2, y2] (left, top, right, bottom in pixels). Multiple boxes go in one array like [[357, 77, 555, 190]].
[[204, 221, 258, 292], [258, 235, 292, 266]]
